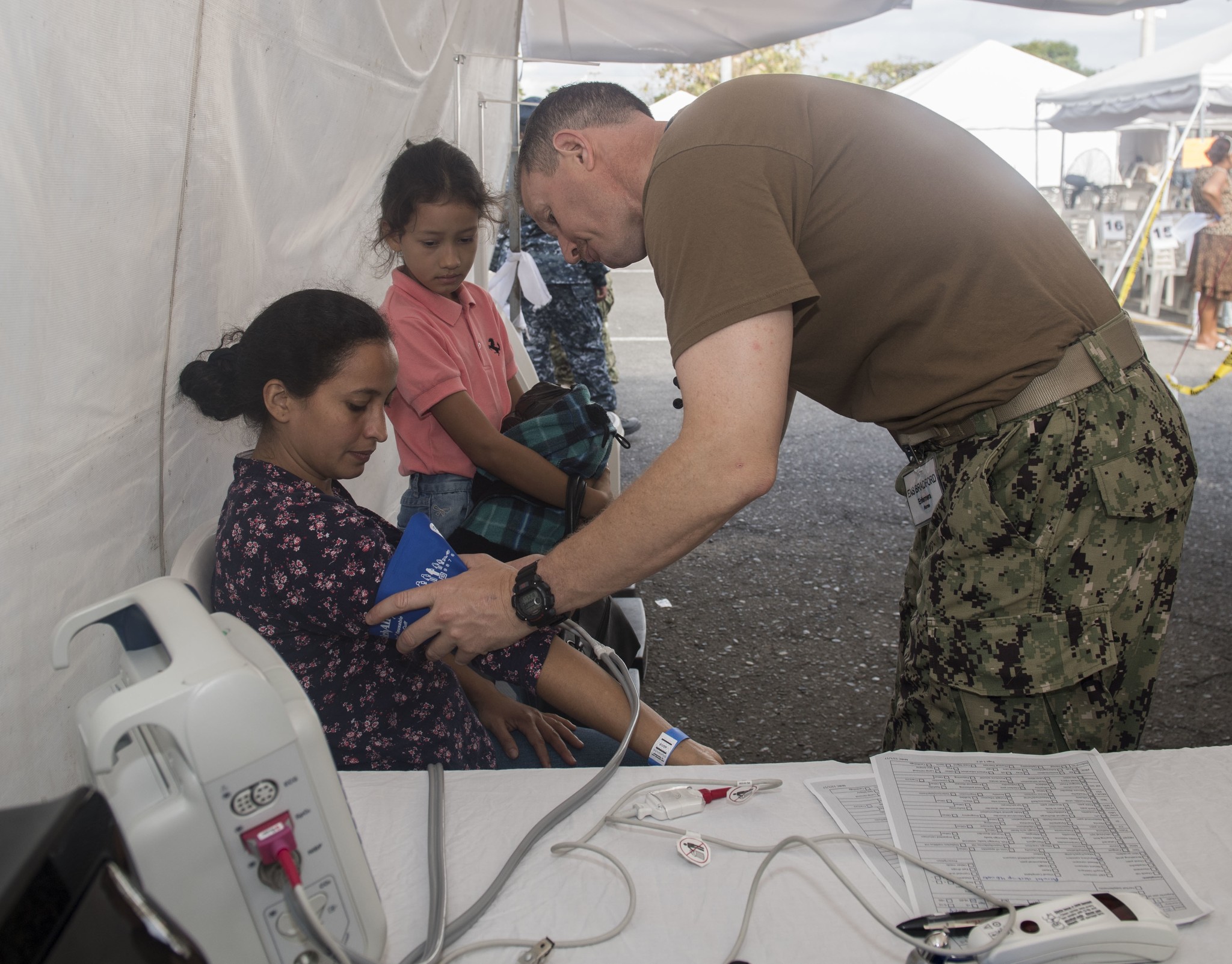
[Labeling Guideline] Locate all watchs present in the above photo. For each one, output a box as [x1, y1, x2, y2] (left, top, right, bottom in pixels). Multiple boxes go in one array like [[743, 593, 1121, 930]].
[[511, 558, 571, 628]]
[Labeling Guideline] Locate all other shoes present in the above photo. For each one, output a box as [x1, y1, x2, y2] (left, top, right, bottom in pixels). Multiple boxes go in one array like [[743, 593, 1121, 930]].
[[1195, 335, 1232, 352], [612, 412, 642, 435]]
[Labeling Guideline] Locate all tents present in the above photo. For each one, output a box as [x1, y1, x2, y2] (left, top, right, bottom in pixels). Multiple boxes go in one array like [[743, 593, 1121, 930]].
[[0, 0, 1232, 819]]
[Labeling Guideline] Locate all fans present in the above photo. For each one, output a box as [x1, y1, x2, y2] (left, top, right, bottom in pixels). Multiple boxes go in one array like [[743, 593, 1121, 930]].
[[1061, 148, 1110, 209]]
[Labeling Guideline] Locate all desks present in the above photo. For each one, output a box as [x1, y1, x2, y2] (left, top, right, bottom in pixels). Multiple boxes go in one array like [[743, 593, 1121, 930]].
[[337, 743, 1232, 963]]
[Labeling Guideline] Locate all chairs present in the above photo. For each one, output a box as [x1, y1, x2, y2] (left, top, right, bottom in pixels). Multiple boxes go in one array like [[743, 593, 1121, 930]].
[[1063, 207, 1196, 319], [494, 298, 626, 499]]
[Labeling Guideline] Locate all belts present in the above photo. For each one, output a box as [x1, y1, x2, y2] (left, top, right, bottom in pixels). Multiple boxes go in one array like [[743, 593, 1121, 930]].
[[898, 313, 1146, 459]]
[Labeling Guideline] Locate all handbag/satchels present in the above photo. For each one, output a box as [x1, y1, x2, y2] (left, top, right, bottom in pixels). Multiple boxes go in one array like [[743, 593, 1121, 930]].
[[558, 472, 641, 681]]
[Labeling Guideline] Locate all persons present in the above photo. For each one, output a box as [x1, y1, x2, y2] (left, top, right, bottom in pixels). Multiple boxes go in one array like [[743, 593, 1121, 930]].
[[491, 210, 643, 436], [178, 287, 724, 773], [371, 138, 615, 560], [546, 260, 621, 392], [366, 73, 1197, 756], [1185, 135, 1232, 352]]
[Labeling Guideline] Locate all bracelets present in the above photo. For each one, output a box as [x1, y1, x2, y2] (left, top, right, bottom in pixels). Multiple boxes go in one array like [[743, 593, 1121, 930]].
[[648, 727, 691, 766]]
